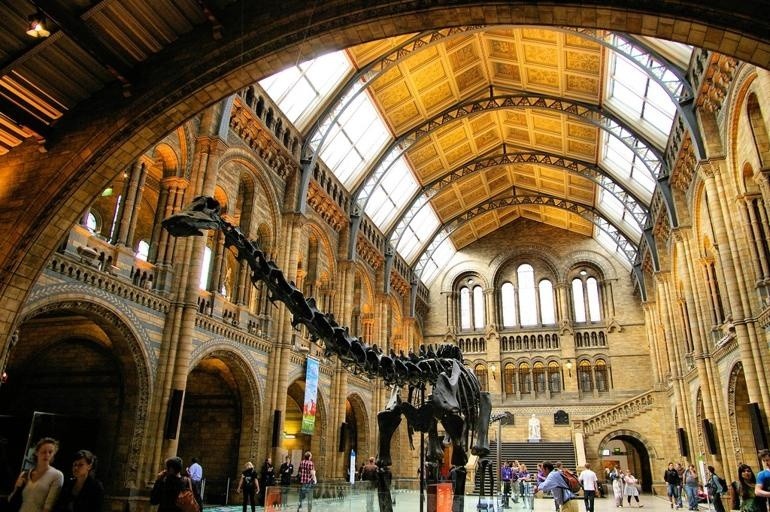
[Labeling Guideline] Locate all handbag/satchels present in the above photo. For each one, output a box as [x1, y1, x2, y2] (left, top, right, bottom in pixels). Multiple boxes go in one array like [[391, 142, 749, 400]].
[[175, 476, 201, 512], [4, 468, 29, 512]]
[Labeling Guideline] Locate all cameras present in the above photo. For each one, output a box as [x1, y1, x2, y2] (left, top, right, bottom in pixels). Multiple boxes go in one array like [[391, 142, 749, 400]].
[[705, 483, 711, 488]]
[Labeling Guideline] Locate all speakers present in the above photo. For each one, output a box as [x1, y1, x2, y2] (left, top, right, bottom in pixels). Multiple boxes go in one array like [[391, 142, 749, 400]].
[[272, 410, 281, 447], [339, 423, 348, 452], [166, 389, 183, 441], [747, 403, 768, 449], [701, 419, 716, 454], [678, 428, 687, 457]]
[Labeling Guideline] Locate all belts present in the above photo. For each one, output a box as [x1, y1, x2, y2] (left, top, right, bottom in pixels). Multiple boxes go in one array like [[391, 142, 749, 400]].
[[563, 496, 576, 504]]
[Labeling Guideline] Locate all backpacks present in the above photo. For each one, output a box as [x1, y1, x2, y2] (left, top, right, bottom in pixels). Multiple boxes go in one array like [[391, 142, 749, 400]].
[[553, 467, 581, 493], [711, 473, 728, 493]]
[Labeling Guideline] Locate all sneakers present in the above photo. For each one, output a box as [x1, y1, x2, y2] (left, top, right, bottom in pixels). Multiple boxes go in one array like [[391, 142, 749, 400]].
[[500, 496, 535, 512], [670, 502, 700, 512], [616, 503, 645, 509]]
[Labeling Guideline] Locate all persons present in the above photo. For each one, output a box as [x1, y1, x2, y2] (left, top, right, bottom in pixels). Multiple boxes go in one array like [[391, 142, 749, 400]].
[[663, 463, 681, 510], [185, 455, 204, 512], [738, 465, 768, 512], [415, 456, 428, 501], [594, 478, 607, 498], [297, 451, 317, 512], [754, 449, 770, 506], [7, 436, 64, 512], [355, 457, 395, 512], [279, 455, 293, 508], [707, 466, 726, 512], [237, 462, 261, 512], [683, 464, 701, 511], [499, 458, 580, 512], [527, 414, 541, 438], [608, 464, 644, 508], [259, 456, 275, 506], [53, 450, 112, 512], [675, 463, 686, 509], [150, 457, 204, 512], [578, 463, 598, 512]]
